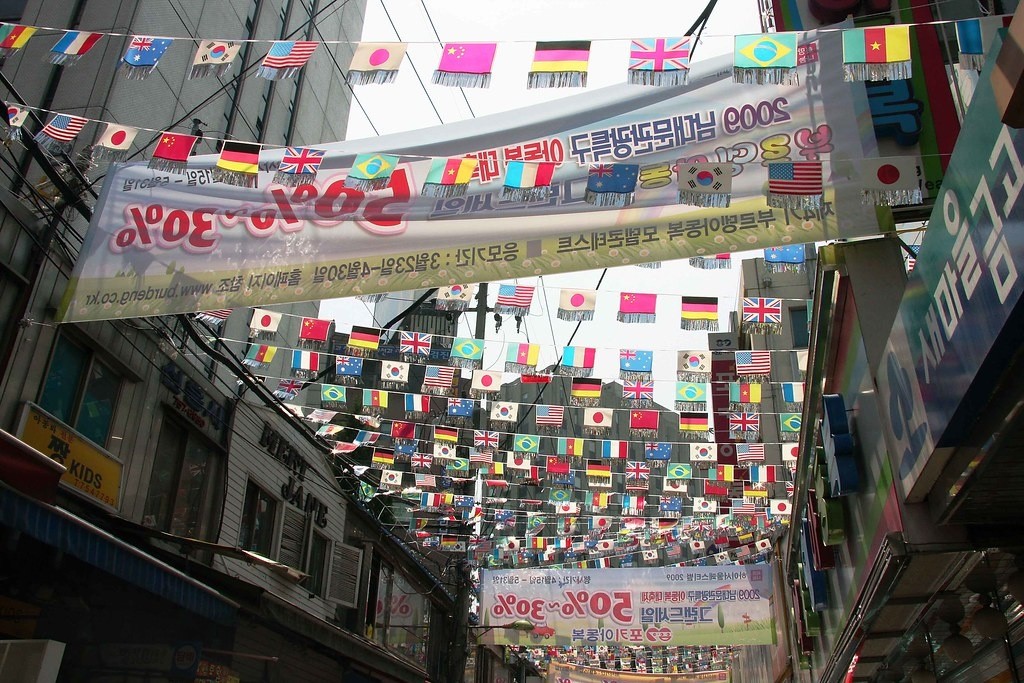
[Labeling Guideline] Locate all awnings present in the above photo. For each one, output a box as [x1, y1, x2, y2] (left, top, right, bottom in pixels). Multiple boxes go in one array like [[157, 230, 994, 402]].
[[0, 481, 240, 632]]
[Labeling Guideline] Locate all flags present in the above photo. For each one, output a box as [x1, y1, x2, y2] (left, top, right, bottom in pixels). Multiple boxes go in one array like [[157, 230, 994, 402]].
[[0, 13, 1004, 209], [193, 240, 809, 673]]
